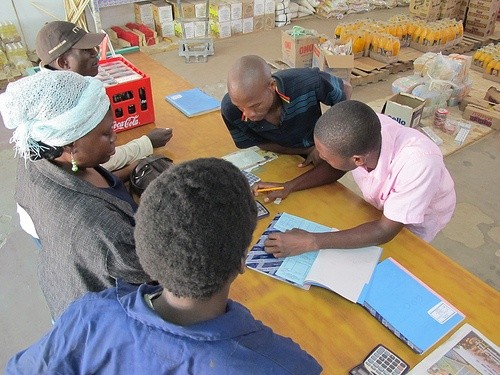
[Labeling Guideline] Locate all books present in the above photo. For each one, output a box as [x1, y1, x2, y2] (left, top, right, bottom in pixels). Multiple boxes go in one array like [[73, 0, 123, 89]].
[[165, 88, 221, 118], [357, 256, 465, 354], [246, 211, 384, 304], [222, 145, 279, 170]]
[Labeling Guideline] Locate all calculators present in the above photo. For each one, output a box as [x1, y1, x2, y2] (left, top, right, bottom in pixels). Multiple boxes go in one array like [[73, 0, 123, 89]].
[[256, 200, 270, 220], [240, 169, 261, 187], [349, 344, 410, 375]]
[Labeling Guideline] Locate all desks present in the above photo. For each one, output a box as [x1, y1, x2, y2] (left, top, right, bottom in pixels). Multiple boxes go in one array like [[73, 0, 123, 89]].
[[98, 50, 500, 375]]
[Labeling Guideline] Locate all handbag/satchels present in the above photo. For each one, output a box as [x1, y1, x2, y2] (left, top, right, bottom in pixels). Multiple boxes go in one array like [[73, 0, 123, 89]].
[[129, 154, 175, 191]]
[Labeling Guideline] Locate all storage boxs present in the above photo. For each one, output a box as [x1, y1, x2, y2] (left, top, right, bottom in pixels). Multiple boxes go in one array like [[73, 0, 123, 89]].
[[0, 0, 500, 262]]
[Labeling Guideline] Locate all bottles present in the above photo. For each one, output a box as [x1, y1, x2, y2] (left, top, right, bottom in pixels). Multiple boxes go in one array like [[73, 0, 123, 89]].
[[114, 88, 147, 118], [0, 21, 27, 69], [434, 108, 448, 128], [472, 42, 500, 77], [319, 14, 463, 56]]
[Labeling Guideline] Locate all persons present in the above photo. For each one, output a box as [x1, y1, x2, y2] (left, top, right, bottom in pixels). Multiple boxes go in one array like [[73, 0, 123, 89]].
[[252, 100, 456, 258], [221, 54, 351, 168], [17, 22, 173, 246], [0, 70, 151, 325], [4, 159, 323, 375]]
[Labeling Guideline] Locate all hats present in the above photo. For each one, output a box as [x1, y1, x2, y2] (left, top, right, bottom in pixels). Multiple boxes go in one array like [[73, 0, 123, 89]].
[[35, 21, 106, 65]]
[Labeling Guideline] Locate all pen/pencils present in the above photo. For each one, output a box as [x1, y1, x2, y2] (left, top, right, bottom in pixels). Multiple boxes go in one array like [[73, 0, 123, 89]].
[[257, 187, 285, 192]]
